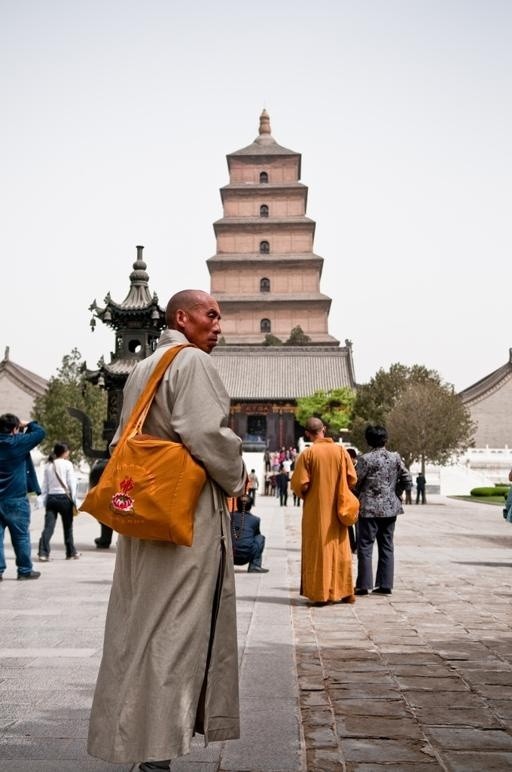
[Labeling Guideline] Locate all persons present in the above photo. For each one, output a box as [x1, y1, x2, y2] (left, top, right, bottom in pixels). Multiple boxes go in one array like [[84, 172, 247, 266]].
[[36, 444, 81, 562], [230, 494, 269, 573], [264, 417, 426, 607], [248, 469, 259, 506], [0, 414, 45, 579], [88, 288, 248, 772], [505, 470, 512, 523]]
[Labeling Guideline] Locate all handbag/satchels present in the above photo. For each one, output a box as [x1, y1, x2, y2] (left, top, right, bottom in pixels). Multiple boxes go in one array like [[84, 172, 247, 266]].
[[72, 504, 79, 516], [334, 487, 361, 527], [80, 429, 207, 549]]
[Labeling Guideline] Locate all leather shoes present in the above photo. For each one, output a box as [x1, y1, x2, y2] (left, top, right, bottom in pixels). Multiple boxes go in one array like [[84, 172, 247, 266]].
[[248, 565, 269, 573]]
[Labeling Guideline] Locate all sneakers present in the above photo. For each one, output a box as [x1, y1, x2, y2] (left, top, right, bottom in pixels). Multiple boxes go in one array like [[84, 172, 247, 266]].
[[17, 570, 41, 580], [38, 553, 52, 562], [372, 587, 393, 597], [65, 551, 81, 561], [95, 536, 111, 549], [351, 587, 369, 596]]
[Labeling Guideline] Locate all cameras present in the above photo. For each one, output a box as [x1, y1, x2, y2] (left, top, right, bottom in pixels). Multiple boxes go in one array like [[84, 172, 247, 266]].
[[18, 427, 24, 433]]
